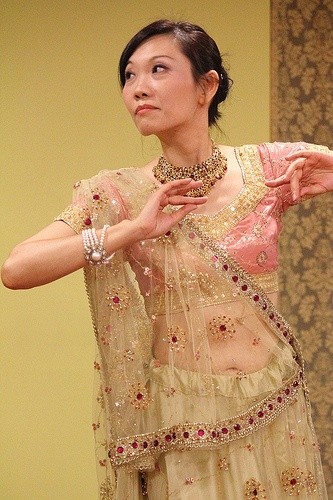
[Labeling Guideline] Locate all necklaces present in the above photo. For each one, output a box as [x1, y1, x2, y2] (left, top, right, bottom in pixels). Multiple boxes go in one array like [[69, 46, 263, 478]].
[[153, 136, 229, 202]]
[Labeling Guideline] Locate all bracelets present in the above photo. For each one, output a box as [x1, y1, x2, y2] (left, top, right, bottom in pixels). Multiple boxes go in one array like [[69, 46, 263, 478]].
[[81, 222, 123, 267]]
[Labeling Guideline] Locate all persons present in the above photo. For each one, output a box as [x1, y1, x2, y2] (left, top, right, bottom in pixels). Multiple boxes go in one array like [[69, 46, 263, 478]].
[[2, 17, 331, 499]]
